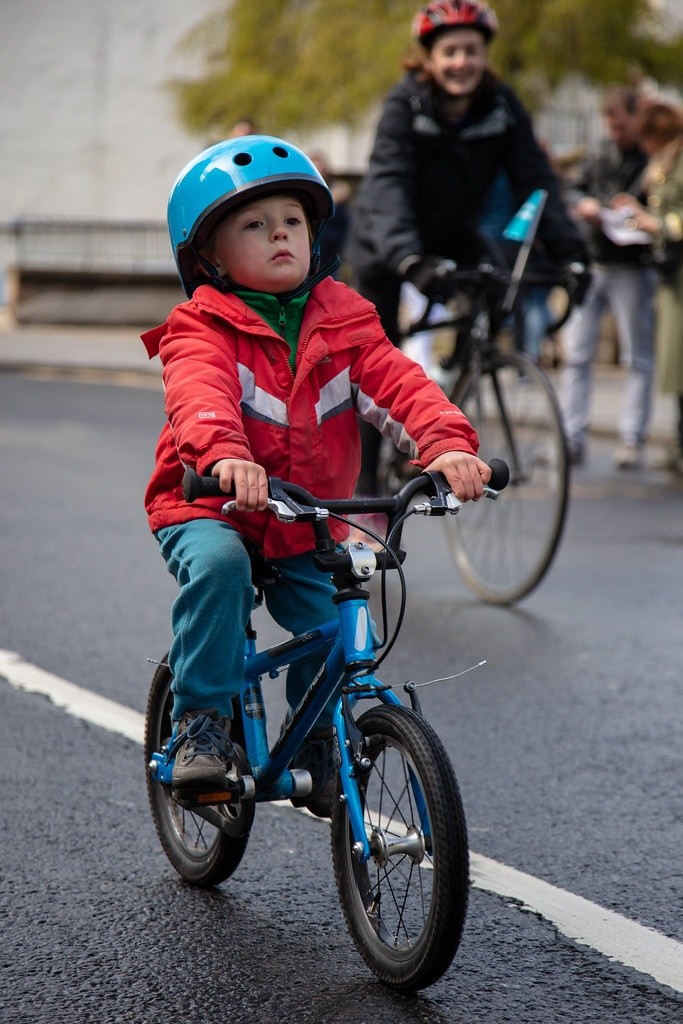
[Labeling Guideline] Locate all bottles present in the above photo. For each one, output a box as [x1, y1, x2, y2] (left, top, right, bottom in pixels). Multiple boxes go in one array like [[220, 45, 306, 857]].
[[430, 359, 454, 392]]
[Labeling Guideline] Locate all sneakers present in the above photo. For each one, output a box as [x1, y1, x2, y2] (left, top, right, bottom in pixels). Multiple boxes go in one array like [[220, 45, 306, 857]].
[[278, 709, 337, 816], [164, 709, 234, 791]]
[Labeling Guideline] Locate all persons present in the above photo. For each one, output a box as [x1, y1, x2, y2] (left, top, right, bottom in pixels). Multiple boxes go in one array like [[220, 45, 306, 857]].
[[532, 88, 682, 470], [529, 301, 561, 367], [229, 119, 254, 137], [310, 157, 350, 256], [348, 0, 594, 555], [142, 134, 491, 817]]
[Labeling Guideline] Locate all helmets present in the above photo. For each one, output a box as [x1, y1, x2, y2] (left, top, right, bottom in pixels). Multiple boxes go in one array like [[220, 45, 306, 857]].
[[168, 135, 342, 301], [411, 0, 499, 53]]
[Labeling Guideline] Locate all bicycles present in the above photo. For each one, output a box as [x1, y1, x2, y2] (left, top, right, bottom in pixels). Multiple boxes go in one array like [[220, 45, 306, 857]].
[[143, 459, 509, 992], [359, 263, 586, 607]]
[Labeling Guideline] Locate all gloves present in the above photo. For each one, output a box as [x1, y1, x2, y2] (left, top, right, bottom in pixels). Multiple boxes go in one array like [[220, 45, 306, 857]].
[[560, 260, 593, 308], [406, 258, 459, 305]]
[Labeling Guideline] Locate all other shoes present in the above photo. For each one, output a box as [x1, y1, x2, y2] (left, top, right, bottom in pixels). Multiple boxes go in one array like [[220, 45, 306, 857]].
[[533, 441, 585, 472], [615, 447, 647, 474]]
